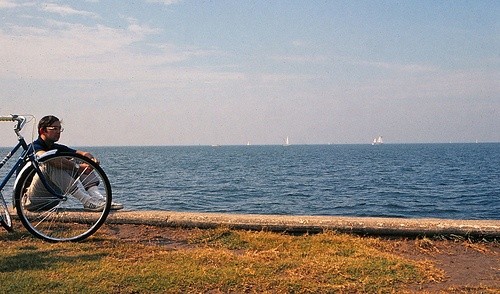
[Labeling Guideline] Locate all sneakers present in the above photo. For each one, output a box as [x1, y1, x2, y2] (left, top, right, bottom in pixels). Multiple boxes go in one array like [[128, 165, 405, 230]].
[[102, 197, 123, 210], [83, 199, 106, 211]]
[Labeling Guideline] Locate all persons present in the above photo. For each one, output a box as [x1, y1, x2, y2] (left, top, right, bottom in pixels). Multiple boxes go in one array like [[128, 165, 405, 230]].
[[15, 115, 123, 212]]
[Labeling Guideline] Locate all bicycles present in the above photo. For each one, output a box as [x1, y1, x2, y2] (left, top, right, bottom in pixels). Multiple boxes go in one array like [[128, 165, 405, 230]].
[[0, 112, 112, 243]]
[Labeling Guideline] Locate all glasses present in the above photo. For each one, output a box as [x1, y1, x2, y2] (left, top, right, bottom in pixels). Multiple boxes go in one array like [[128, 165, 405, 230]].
[[44, 126, 63, 132]]
